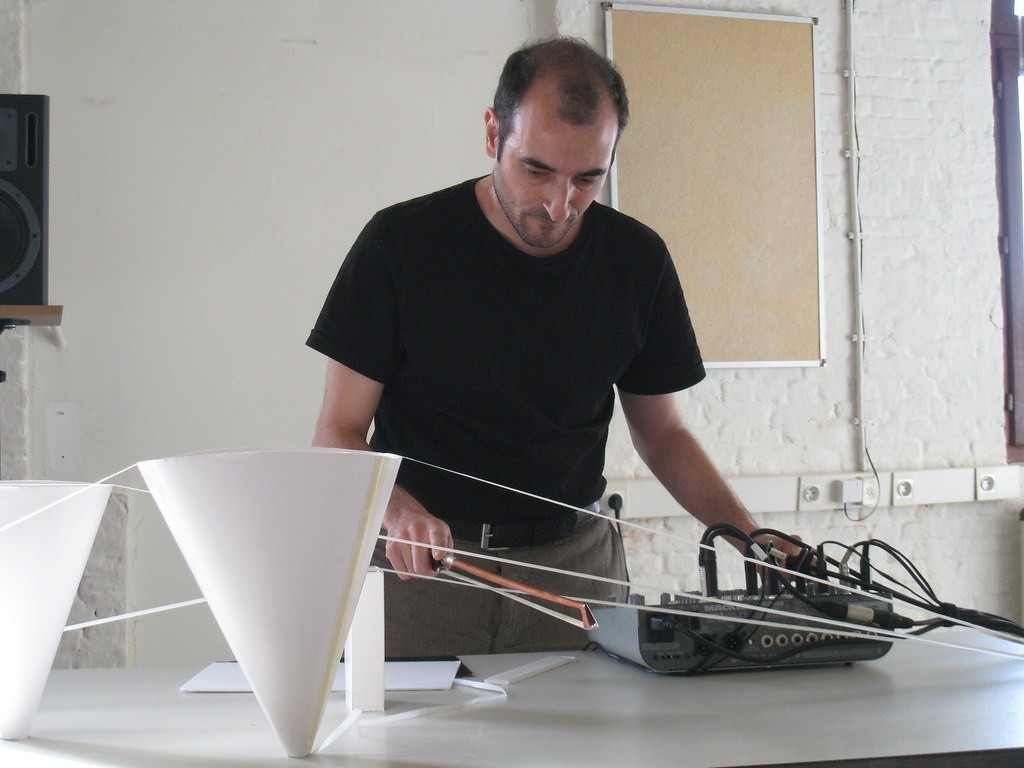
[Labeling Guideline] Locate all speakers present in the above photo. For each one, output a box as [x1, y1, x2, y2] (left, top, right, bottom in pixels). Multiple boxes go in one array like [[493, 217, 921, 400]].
[[0, 93, 50, 307]]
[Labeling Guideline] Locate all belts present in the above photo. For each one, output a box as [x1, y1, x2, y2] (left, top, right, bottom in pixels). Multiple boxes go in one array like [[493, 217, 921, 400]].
[[442, 501, 602, 552]]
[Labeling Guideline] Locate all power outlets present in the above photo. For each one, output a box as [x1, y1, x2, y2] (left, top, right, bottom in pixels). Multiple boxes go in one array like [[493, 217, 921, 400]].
[[803, 482, 822, 504], [604, 491, 625, 512], [895, 478, 913, 499], [979, 474, 996, 494]]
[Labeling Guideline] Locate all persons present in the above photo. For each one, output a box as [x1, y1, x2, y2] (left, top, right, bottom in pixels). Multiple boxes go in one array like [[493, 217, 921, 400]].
[[306, 34, 817, 657]]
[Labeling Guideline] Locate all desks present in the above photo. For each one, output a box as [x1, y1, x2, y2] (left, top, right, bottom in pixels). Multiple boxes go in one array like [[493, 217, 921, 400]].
[[0, 613, 1024, 768]]
